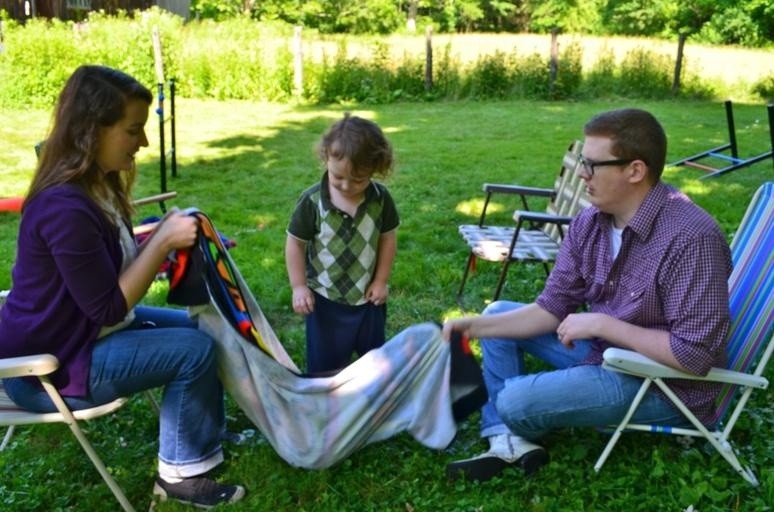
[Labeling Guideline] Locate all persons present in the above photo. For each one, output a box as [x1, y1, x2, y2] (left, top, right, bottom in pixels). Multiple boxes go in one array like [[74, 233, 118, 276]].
[[285, 115, 397, 379], [0, 64, 246, 506], [441, 107, 732, 480]]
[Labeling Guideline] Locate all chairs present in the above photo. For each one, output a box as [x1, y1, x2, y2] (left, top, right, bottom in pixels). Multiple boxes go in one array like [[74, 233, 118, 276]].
[[592, 181, 774, 488], [132, 190, 177, 242], [1, 291, 161, 512], [455, 140, 590, 302]]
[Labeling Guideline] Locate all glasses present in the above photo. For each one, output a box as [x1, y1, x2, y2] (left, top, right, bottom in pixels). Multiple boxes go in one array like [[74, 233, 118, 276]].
[[577, 154, 632, 176]]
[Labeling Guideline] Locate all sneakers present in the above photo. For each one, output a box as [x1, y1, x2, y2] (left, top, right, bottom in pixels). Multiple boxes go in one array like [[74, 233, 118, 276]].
[[220, 431, 247, 446], [153, 473, 245, 509], [446, 442, 551, 484]]
